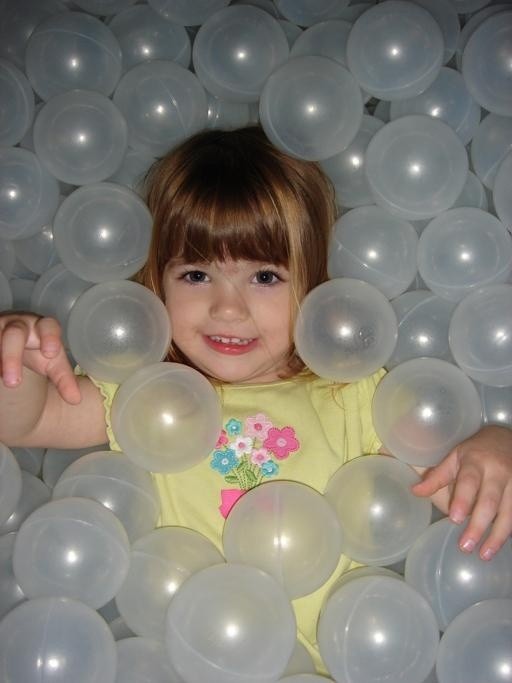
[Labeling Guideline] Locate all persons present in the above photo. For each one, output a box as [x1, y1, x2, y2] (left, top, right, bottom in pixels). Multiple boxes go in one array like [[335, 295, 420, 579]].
[[0, 124, 512, 680]]
